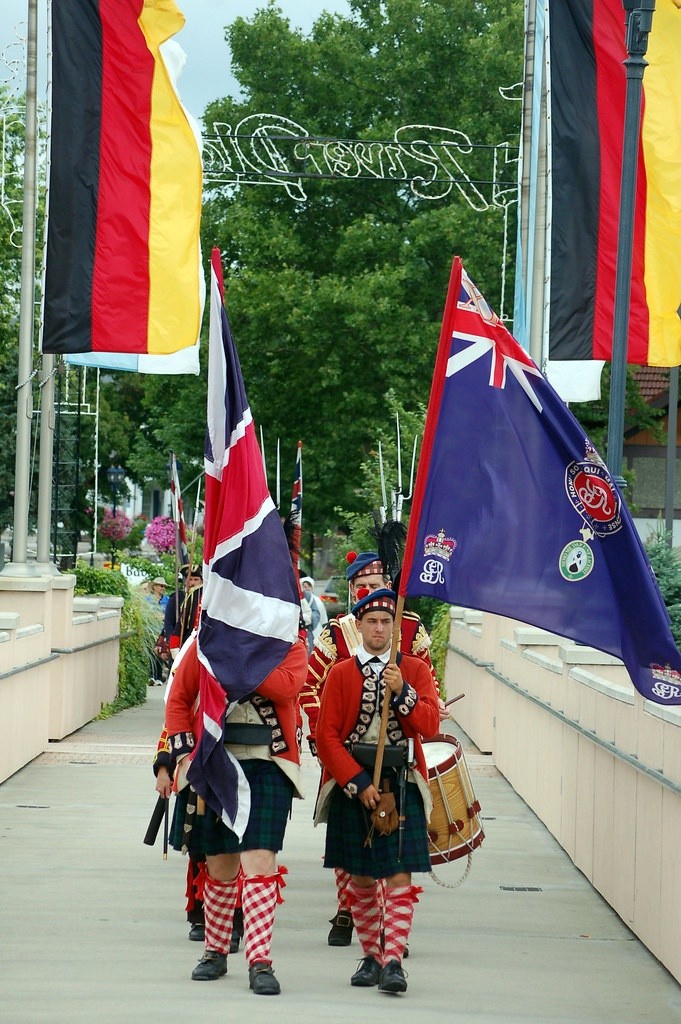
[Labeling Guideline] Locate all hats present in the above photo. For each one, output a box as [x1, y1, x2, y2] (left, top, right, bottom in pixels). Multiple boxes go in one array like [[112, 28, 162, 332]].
[[351, 588, 397, 620], [180, 564, 202, 579], [299, 576, 314, 589], [346, 520, 408, 582], [148, 577, 168, 592]]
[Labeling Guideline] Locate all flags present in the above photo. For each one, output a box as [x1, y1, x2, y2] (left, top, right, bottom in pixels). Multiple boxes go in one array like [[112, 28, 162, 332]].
[[291, 441, 302, 598], [399, 256, 681, 706], [41, 0, 205, 376], [185, 248, 302, 843], [172, 454, 189, 564], [513, 0, 681, 403]]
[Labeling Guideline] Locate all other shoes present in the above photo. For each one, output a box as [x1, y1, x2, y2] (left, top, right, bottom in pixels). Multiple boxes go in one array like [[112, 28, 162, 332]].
[[148, 678, 163, 686]]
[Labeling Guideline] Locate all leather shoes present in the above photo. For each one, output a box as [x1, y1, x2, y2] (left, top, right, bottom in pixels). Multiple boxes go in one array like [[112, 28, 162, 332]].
[[192, 951, 228, 981], [189, 922, 205, 941], [249, 964, 281, 994], [230, 931, 240, 952], [351, 956, 407, 992], [327, 911, 354, 946]]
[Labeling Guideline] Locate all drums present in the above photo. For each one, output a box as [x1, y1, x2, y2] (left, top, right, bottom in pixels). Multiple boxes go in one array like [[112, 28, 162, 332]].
[[421, 734, 485, 865]]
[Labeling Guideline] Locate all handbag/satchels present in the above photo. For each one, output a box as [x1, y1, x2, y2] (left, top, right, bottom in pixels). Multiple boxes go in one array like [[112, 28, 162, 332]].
[[351, 743, 403, 790]]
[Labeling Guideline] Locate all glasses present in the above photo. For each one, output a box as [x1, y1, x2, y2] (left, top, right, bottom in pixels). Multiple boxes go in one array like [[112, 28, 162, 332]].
[[160, 585, 166, 589]]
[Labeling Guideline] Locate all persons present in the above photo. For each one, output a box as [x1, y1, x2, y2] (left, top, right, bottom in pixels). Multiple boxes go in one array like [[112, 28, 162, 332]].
[[145, 553, 451, 952], [164, 631, 307, 995], [315, 587, 440, 993]]
